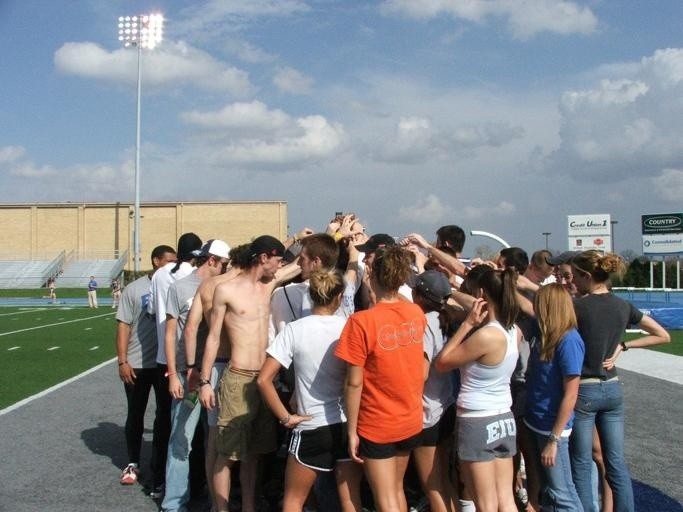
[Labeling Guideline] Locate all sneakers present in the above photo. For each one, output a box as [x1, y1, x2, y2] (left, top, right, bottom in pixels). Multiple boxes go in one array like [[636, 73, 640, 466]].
[[121, 464, 138, 484], [150, 484, 164, 499]]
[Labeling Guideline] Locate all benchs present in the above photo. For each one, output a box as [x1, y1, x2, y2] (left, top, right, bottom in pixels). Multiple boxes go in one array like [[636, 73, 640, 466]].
[[0, 259, 117, 288]]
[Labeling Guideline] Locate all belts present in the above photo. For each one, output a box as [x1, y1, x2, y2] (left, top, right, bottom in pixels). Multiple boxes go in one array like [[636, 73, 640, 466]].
[[578, 376, 618, 384]]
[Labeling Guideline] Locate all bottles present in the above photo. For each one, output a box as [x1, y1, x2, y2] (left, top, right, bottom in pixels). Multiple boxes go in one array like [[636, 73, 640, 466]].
[[183, 381, 200, 409]]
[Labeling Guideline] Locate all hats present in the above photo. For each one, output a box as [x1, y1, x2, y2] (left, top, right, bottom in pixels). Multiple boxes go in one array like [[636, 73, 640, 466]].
[[354, 234, 394, 252], [544, 251, 578, 266], [246, 235, 284, 259], [405, 271, 452, 304], [189, 239, 230, 259]]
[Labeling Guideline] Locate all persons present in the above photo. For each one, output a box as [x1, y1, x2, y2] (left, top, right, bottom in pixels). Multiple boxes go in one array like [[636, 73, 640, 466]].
[[116, 211, 670, 510], [41, 278, 56, 304], [111, 278, 123, 309], [87, 275, 98, 309]]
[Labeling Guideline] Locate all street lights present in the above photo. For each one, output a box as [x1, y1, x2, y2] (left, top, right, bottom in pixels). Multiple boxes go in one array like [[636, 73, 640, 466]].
[[116, 10, 167, 281], [541, 231, 551, 254], [608, 220, 618, 256]]
